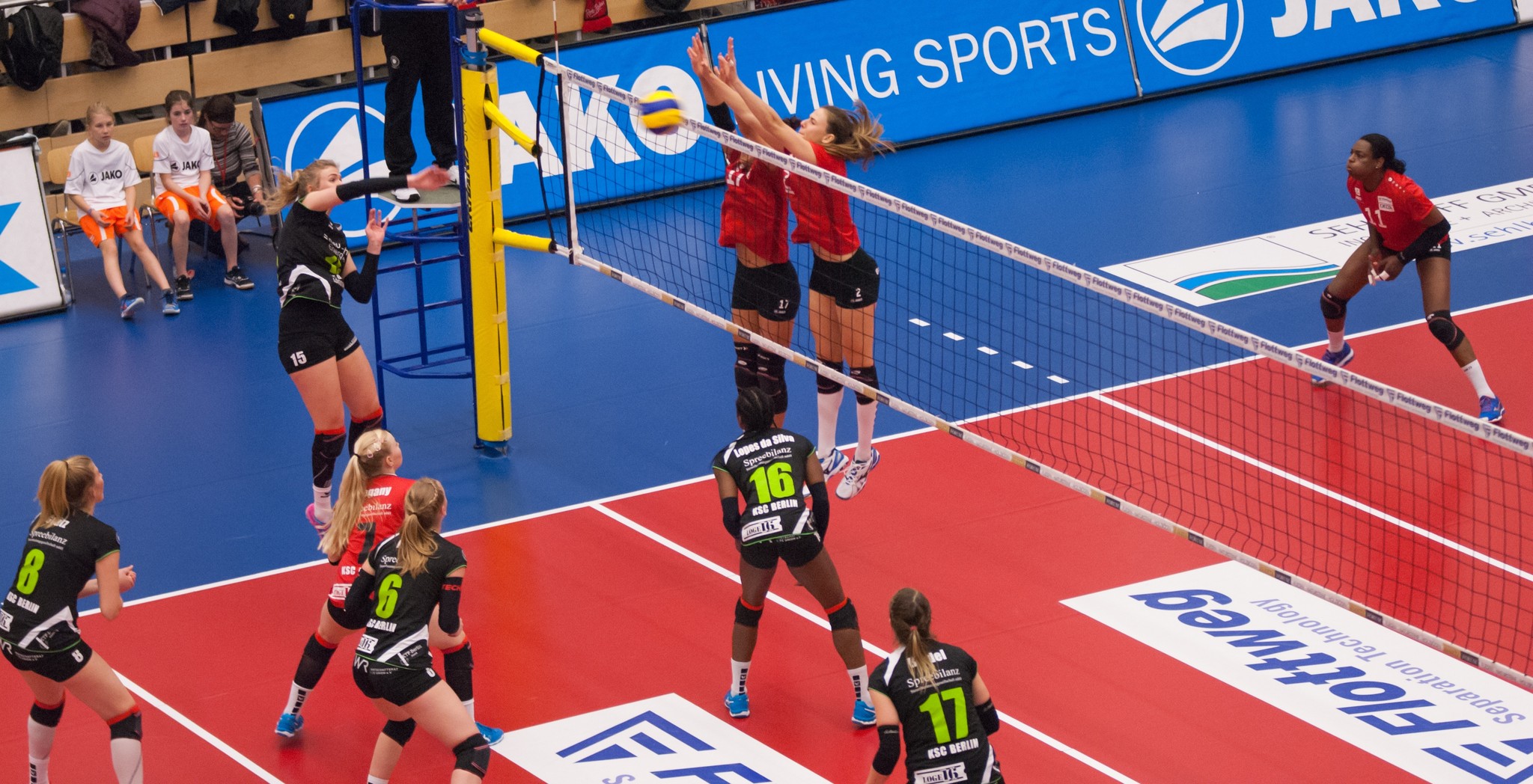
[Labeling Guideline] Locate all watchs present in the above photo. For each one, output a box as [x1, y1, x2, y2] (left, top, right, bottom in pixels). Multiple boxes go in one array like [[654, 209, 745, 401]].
[[250, 185, 263, 194]]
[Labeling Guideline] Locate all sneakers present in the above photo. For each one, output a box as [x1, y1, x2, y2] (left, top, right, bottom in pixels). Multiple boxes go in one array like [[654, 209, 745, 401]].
[[224, 266, 255, 290], [1312, 342, 1354, 384], [442, 165, 461, 191], [1476, 395, 1505, 422], [176, 274, 194, 300], [120, 294, 146, 317], [851, 700, 877, 728], [834, 446, 879, 500], [159, 289, 180, 313], [276, 714, 305, 738], [725, 689, 751, 718], [390, 187, 420, 202], [803, 449, 849, 501], [476, 722, 503, 748]]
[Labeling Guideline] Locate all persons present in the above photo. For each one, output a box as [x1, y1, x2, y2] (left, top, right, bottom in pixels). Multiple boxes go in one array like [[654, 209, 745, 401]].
[[64, 101, 180, 319], [277, 159, 451, 527], [379, 0, 467, 202], [1310, 133, 1507, 424], [0, 456, 143, 784], [686, 32, 881, 500], [152, 90, 266, 300], [865, 588, 1005, 784], [274, 430, 490, 784], [709, 388, 880, 726]]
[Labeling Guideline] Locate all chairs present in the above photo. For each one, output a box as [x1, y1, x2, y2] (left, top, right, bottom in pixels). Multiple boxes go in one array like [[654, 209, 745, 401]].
[[46, 132, 209, 302]]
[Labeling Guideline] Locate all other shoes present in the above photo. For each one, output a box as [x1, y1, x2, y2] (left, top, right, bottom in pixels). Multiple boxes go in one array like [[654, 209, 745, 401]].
[[305, 502, 329, 541]]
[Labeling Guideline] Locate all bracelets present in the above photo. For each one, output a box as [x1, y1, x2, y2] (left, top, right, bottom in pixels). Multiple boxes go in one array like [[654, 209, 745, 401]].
[[87, 208, 94, 215]]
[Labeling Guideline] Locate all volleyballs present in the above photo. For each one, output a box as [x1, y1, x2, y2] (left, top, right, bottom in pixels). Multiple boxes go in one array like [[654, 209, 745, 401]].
[[637, 90, 686, 135]]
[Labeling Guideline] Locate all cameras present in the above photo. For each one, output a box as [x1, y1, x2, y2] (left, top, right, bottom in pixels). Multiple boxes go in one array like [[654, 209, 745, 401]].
[[233, 196, 266, 219]]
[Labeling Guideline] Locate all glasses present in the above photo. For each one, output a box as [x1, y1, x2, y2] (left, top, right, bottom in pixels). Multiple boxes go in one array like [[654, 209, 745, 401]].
[[211, 121, 236, 131]]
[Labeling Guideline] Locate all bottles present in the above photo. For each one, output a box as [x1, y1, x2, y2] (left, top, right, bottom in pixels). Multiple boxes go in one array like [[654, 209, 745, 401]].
[[60, 267, 72, 306]]
